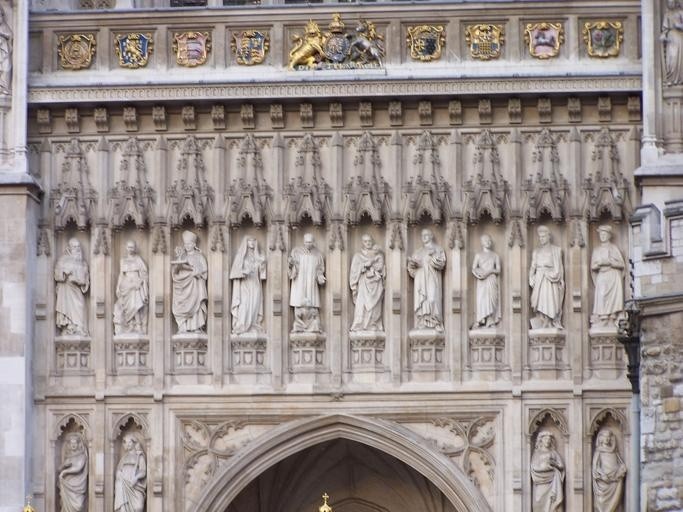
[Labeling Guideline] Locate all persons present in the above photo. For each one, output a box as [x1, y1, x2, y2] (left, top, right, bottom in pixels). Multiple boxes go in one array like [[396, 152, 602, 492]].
[[171, 231, 208, 335], [114, 434, 146, 512], [590, 224, 625, 320], [593, 430, 627, 512], [57, 432, 89, 512], [472, 234, 501, 330], [408, 227, 446, 332], [529, 225, 565, 332], [54, 236, 91, 334], [530, 432, 565, 512], [113, 238, 149, 337], [287, 233, 327, 333], [230, 234, 267, 336], [350, 233, 386, 330]]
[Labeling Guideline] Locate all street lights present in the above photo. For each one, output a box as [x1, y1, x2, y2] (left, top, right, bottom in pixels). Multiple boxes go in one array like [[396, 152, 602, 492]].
[[614, 296, 645, 512]]
